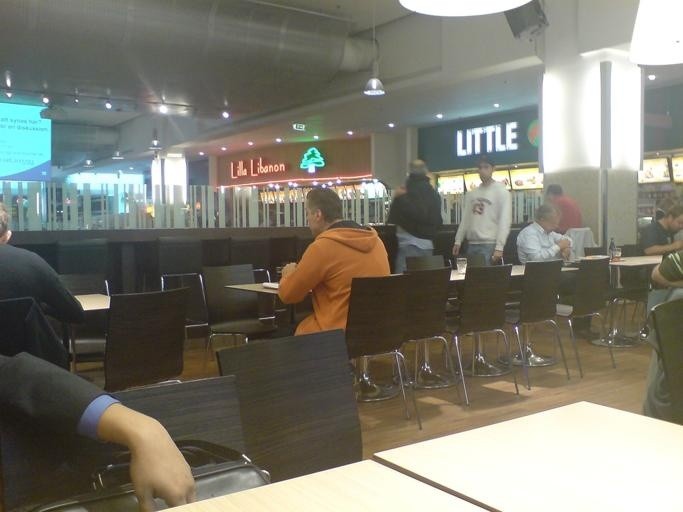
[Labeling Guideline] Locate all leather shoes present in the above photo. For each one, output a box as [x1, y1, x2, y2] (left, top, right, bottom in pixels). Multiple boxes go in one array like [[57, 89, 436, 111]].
[[570, 327, 600, 339]]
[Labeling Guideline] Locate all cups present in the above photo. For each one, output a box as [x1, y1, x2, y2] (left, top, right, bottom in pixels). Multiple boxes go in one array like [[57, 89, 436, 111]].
[[456, 258, 467, 275], [613, 248, 621, 261], [275, 267, 283, 287]]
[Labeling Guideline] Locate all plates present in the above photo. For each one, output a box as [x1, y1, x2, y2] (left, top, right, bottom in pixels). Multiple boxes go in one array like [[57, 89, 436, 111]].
[[263, 283, 278, 289]]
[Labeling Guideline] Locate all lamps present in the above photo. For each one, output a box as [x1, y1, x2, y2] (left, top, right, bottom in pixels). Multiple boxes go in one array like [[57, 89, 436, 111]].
[[0, 84, 229, 170], [363, 0, 385, 96]]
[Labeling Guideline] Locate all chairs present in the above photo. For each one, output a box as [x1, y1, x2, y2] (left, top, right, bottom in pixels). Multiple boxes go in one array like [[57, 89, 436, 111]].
[[106, 287, 190, 390], [405, 268, 462, 413], [68, 375, 246, 500], [503, 259, 572, 388], [505, 224, 539, 266], [215, 328, 364, 487], [331, 272, 413, 424], [610, 262, 652, 349], [201, 263, 275, 340], [155, 270, 212, 349], [455, 266, 521, 402], [555, 257, 619, 379], [0, 299, 74, 371]]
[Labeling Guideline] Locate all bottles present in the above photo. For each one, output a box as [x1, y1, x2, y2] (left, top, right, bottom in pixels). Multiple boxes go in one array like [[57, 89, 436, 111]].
[[608, 237, 616, 260]]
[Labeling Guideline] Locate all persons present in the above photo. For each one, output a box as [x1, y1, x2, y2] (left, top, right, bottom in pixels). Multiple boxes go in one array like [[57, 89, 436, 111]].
[[547, 183, 583, 235], [271, 188, 392, 338], [638, 197, 683, 426], [452, 157, 513, 266], [0, 203, 84, 322], [0, 352, 195, 511], [388, 158, 442, 273], [516, 203, 601, 339]]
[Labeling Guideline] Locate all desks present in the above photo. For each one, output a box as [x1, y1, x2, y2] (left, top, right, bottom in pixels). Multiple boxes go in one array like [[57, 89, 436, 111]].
[[163, 398, 682, 512], [223, 250, 665, 300], [71, 288, 115, 314]]
